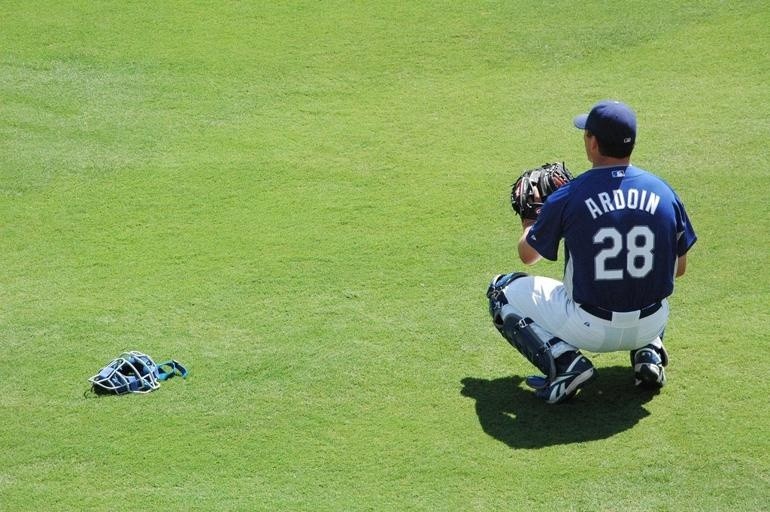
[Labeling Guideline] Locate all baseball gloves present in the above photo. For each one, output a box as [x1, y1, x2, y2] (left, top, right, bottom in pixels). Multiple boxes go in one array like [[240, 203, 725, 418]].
[[511, 161, 575, 221]]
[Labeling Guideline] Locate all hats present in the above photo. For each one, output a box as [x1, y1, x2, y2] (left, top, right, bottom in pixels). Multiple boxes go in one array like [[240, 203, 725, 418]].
[[572, 103, 637, 144]]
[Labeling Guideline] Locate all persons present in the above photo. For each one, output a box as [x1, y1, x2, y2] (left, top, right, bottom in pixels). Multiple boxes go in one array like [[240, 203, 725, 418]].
[[486, 101, 697, 406]]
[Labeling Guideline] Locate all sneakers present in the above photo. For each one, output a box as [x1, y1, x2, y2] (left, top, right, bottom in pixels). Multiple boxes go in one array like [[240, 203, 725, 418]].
[[524, 350, 597, 404], [627, 344, 671, 388]]
